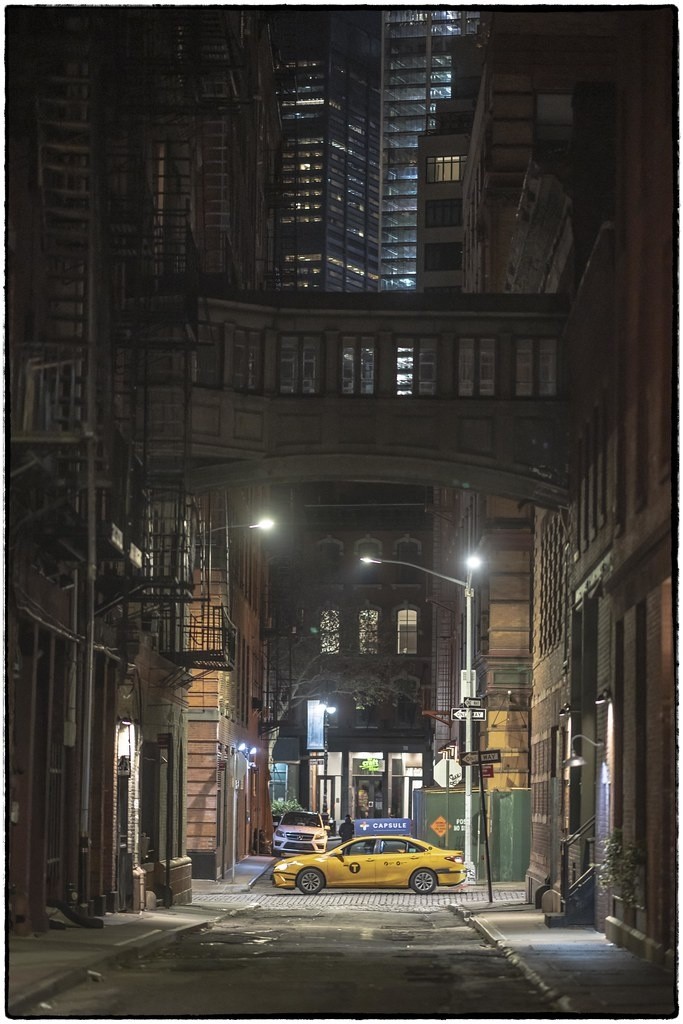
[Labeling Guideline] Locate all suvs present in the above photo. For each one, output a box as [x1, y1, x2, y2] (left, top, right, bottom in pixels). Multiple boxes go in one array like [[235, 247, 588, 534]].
[[272, 811, 331, 857]]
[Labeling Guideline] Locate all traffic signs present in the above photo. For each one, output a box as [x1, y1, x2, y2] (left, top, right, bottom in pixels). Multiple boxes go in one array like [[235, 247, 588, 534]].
[[459, 749, 501, 767], [451, 697, 486, 721]]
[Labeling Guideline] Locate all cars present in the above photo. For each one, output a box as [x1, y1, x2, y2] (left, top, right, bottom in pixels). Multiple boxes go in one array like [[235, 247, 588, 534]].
[[271, 836, 466, 894]]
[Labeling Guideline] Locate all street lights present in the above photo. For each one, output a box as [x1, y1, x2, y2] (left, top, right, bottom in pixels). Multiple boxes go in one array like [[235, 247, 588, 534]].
[[360, 554, 482, 862]]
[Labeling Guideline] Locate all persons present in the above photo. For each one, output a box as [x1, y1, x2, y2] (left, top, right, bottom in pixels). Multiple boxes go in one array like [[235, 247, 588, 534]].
[[338, 816, 354, 855], [365, 842, 373, 854]]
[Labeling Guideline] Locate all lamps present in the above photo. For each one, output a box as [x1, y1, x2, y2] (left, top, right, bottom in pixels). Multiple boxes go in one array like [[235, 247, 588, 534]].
[[563, 735, 605, 766]]
